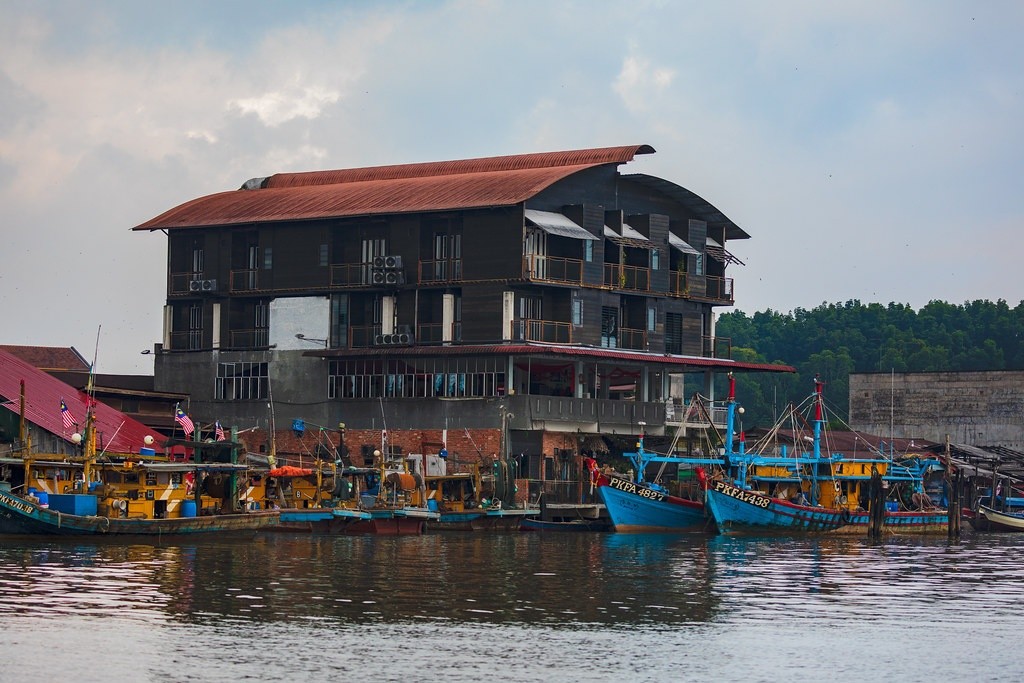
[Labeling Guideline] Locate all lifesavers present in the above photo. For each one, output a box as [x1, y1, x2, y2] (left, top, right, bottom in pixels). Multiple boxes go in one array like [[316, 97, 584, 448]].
[[834, 481, 840, 491], [841, 510, 850, 523], [841, 495, 848, 504], [945, 466, 957, 480]]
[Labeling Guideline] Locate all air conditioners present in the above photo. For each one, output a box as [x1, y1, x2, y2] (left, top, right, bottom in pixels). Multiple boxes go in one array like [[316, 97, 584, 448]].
[[376, 324, 412, 345], [201, 279, 216, 291], [190, 281, 201, 291], [373, 272, 385, 283], [386, 272, 404, 284], [385, 256, 401, 268], [373, 256, 384, 268]]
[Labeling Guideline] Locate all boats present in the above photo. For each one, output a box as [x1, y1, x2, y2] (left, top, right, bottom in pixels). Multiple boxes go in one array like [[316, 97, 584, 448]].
[[0, 321, 1023, 540]]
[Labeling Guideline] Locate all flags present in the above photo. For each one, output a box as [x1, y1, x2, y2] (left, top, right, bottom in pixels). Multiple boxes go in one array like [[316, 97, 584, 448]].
[[61, 399, 77, 431], [216, 420, 226, 441], [175, 407, 194, 435], [995, 480, 1002, 495]]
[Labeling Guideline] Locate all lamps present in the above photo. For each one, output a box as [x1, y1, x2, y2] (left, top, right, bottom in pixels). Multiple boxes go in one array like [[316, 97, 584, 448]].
[[141, 350, 155, 354]]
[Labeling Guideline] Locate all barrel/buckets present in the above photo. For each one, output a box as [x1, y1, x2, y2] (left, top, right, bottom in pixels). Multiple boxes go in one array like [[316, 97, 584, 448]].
[[139, 447, 155, 456], [427, 500, 437, 511], [34, 492, 48, 505], [884, 501, 898, 512], [28, 487, 37, 495], [180, 499, 196, 517]]
